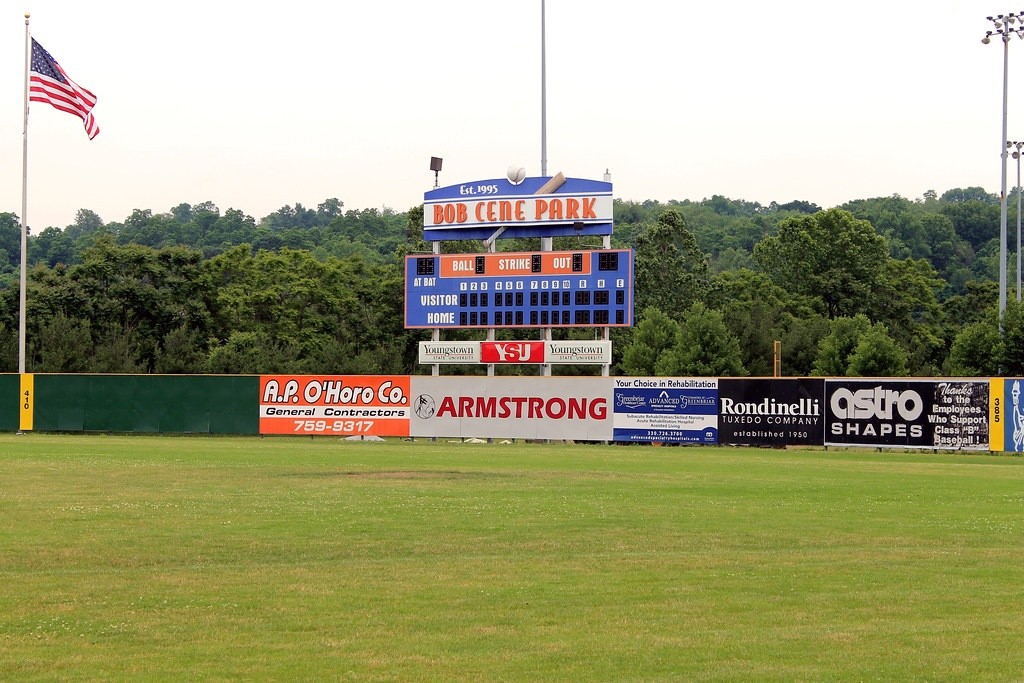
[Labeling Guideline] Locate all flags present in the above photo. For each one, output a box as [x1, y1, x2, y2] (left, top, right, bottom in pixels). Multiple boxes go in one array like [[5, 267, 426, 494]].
[[29, 37, 100, 140]]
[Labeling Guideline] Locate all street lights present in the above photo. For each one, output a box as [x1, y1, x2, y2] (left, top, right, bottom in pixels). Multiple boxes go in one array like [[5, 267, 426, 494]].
[[980, 10, 1023, 376], [998, 139, 1024, 321]]
